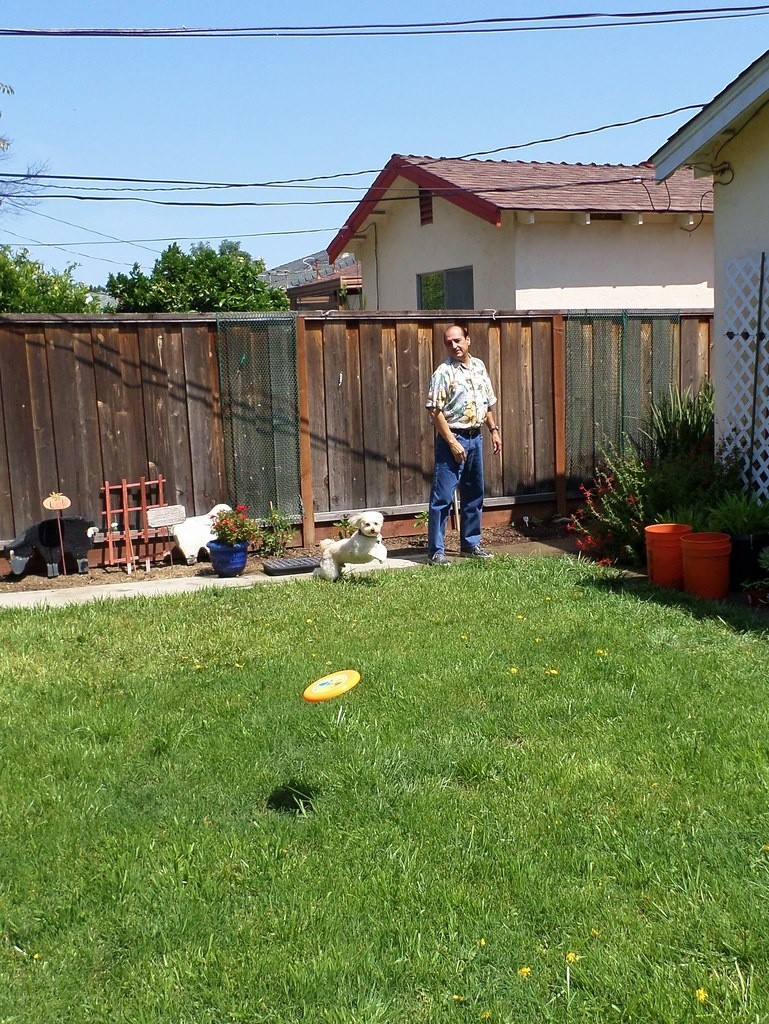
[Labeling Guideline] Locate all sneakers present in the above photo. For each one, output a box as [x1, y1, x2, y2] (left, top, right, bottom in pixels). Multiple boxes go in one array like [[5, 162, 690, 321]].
[[427, 554, 451, 566], [460, 545, 494, 558]]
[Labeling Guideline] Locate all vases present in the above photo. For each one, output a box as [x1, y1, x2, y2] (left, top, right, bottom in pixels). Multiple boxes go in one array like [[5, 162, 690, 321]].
[[206, 539, 249, 578]]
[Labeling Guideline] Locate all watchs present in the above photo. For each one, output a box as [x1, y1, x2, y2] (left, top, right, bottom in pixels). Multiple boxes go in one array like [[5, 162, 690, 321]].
[[490, 426, 498, 433]]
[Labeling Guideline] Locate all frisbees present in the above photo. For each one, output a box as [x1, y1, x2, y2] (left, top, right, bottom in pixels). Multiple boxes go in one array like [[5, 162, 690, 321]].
[[304, 668, 361, 702]]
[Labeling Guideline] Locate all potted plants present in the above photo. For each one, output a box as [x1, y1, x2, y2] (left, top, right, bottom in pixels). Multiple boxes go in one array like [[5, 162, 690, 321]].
[[704, 485, 769, 590], [741, 578, 768, 608]]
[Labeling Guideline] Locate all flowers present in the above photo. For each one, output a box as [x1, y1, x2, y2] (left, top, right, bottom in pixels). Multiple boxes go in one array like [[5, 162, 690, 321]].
[[208, 505, 264, 551]]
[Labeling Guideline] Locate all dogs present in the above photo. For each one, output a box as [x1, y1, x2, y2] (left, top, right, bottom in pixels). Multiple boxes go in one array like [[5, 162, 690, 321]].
[[312, 510, 387, 581]]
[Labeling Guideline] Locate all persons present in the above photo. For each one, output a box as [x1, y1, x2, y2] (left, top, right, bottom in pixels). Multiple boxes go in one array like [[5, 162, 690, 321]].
[[425, 325, 502, 566]]
